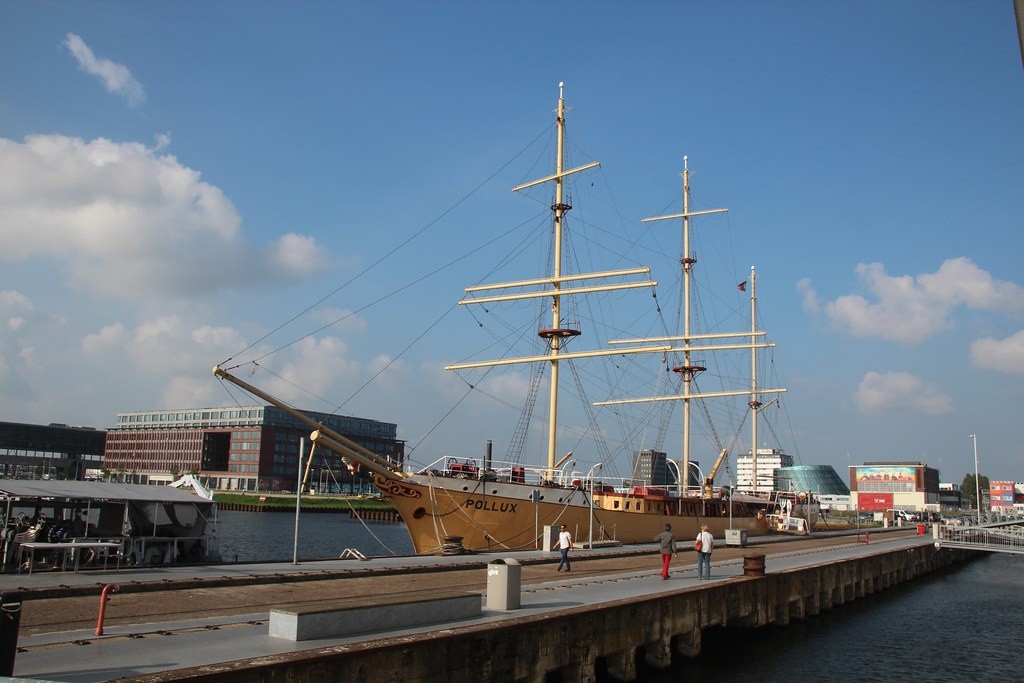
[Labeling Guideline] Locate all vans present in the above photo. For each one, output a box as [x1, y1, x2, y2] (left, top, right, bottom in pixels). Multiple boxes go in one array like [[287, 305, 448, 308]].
[[887, 508, 917, 523]]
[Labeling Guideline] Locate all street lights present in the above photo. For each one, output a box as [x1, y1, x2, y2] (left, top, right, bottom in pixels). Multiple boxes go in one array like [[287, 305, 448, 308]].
[[969, 433, 981, 526]]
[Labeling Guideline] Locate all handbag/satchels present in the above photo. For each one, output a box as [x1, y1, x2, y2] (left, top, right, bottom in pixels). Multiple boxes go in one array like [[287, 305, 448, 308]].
[[694, 541, 702, 551]]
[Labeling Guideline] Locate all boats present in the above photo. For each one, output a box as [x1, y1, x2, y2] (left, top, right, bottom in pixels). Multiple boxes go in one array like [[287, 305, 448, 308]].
[[0, 476, 224, 576]]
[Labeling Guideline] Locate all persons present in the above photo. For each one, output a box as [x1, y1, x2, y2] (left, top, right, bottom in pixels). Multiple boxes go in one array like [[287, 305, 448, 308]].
[[695, 525, 714, 580], [655, 524, 678, 580], [553, 524, 573, 572]]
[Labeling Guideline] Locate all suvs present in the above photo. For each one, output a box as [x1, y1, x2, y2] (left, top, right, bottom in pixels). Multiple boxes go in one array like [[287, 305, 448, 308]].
[[916, 510, 944, 522]]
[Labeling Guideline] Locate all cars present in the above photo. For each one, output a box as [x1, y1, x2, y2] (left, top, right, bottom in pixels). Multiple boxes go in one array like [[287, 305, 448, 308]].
[[859, 513, 874, 522]]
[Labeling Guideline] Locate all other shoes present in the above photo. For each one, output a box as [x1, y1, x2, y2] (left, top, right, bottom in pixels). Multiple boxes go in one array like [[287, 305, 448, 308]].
[[556, 568, 560, 571], [565, 569, 570, 571], [668, 575, 671, 577], [662, 577, 666, 580], [704, 576, 709, 580], [697, 577, 701, 580]]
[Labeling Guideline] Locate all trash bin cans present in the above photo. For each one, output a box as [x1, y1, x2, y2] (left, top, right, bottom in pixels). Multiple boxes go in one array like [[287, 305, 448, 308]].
[[486, 558, 522, 610]]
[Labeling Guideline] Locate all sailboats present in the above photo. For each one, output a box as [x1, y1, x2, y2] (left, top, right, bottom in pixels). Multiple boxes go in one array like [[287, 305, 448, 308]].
[[212, 78, 832, 555]]
